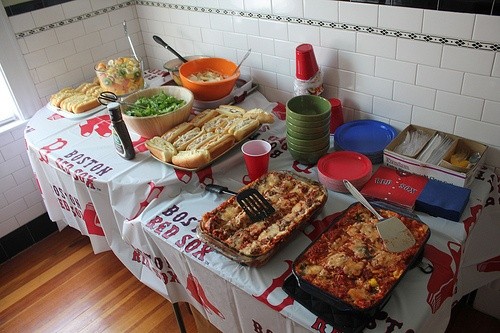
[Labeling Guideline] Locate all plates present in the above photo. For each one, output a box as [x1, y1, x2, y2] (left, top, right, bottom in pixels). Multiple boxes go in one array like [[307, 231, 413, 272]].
[[317, 119, 398, 195], [149, 124, 262, 170], [49, 84, 148, 119]]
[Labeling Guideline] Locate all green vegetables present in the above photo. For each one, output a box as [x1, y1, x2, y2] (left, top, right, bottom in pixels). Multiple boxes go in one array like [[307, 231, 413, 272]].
[[127, 91, 186, 117]]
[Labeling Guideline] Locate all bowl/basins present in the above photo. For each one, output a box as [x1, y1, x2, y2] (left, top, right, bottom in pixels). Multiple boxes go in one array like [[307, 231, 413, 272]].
[[118, 85, 194, 137], [163, 56, 209, 86], [179, 57, 241, 101], [285, 95, 332, 165], [94, 56, 145, 102]]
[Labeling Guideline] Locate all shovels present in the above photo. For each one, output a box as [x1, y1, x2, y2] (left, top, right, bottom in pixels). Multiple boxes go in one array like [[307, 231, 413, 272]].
[[204, 183, 275, 223], [342, 179, 416, 253]]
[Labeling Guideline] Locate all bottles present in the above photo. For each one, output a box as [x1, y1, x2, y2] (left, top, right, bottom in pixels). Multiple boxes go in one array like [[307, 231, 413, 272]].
[[107, 102, 135, 161]]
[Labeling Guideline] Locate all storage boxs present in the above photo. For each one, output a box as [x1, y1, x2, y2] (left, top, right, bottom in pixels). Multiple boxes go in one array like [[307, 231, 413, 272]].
[[384, 123, 487, 188]]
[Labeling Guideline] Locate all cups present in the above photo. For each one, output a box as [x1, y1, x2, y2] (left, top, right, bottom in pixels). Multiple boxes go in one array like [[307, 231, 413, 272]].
[[327, 98, 344, 136], [295, 43, 319, 80], [241, 140, 272, 181]]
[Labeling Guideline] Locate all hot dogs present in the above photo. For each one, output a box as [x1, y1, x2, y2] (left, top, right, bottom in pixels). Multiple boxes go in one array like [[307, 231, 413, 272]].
[[50, 79, 106, 114], [145, 104, 274, 167]]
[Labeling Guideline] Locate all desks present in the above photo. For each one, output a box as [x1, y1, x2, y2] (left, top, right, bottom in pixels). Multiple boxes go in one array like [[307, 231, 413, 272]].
[[23, 69, 500, 333]]
